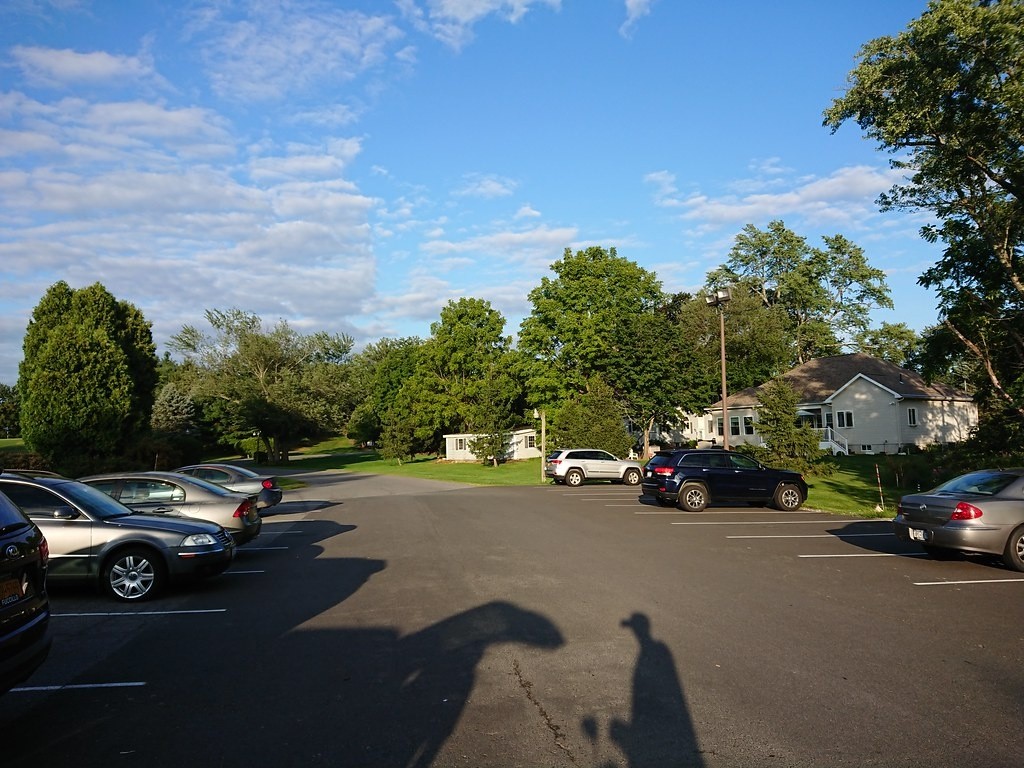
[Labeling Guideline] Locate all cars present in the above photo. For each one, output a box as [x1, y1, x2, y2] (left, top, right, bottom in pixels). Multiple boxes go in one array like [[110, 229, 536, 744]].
[[177, 464, 283, 511], [75, 472, 263, 547], [891, 467, 1024, 572]]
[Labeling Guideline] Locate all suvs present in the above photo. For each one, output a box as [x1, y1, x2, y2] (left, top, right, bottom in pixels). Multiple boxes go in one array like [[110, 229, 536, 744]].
[[0, 491, 56, 698], [544, 448, 644, 487], [641, 449, 808, 513], [0, 469, 240, 603]]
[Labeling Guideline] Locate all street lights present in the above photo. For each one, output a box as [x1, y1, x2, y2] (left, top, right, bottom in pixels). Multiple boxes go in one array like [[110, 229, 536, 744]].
[[704, 286, 733, 451]]
